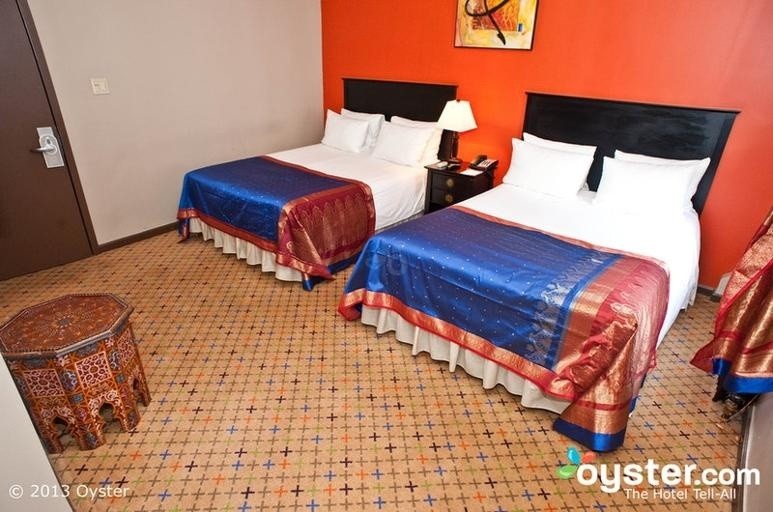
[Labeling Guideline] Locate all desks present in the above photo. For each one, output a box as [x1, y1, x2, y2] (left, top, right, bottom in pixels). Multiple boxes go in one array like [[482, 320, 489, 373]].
[[0, 292, 152, 453]]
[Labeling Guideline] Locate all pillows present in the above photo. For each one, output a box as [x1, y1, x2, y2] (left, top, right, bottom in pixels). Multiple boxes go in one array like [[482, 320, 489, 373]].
[[503, 137, 594, 200], [391, 115, 444, 165], [372, 122, 433, 167], [523, 132, 597, 157], [340, 107, 385, 153], [321, 108, 369, 154], [592, 149, 710, 221]]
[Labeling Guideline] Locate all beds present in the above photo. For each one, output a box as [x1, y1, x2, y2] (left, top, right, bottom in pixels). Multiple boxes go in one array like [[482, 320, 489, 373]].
[[339, 90, 741, 452], [176, 77, 459, 292]]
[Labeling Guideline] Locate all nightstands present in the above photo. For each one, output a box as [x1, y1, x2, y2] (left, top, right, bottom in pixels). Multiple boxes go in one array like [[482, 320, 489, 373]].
[[424, 160, 495, 214]]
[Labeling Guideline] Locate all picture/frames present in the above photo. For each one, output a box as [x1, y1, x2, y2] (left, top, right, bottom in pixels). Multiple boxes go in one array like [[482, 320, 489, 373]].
[[454, 0, 539, 51]]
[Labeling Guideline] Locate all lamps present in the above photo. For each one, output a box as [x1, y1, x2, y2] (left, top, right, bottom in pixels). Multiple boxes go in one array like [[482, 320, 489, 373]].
[[437, 99, 477, 164]]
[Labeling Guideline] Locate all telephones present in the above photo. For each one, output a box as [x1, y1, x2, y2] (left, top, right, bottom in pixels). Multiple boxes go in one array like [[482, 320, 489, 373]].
[[468, 155, 498, 170]]
